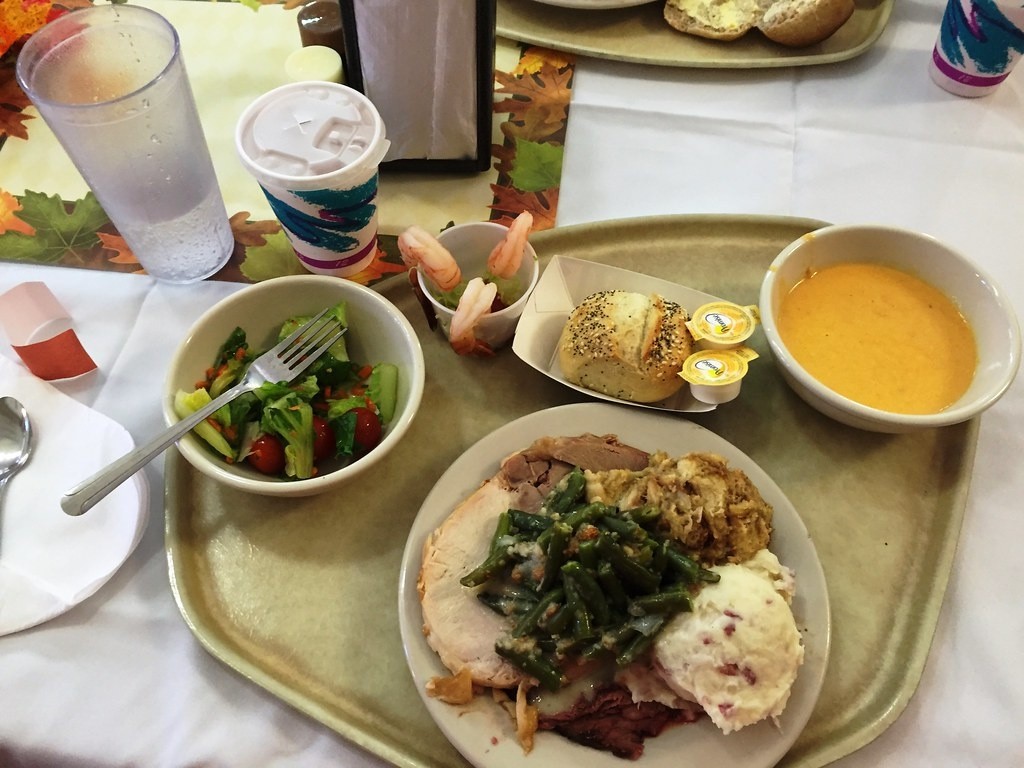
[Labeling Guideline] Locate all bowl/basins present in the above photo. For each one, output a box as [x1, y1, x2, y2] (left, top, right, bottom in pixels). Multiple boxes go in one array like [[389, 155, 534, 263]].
[[759, 223, 1021, 433], [162, 275, 427, 499]]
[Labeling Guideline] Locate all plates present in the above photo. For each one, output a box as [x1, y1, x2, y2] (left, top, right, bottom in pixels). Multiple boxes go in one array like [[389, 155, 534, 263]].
[[496, 0, 894, 70], [397, 403, 831, 768]]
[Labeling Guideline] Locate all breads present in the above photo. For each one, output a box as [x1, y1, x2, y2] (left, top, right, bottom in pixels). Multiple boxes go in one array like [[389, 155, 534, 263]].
[[559, 290, 695, 403], [662, 0, 853, 48]]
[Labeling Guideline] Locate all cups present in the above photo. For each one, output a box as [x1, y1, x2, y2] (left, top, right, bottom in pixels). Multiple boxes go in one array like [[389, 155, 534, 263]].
[[16, 4, 235, 286], [416, 221, 539, 354], [235, 81, 392, 277], [929, 0, 1024, 98]]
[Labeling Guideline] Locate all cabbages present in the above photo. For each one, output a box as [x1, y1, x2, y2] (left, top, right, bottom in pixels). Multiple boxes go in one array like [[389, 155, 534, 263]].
[[174, 302, 397, 480]]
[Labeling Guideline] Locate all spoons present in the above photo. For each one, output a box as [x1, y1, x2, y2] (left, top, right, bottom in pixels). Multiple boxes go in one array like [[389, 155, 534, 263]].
[[0, 396, 32, 559]]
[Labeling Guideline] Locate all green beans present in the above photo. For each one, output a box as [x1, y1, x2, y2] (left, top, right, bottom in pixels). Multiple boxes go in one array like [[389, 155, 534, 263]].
[[460, 472, 720, 697]]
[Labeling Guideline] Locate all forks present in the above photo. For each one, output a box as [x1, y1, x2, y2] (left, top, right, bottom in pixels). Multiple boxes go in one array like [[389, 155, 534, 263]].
[[59, 307, 348, 516]]
